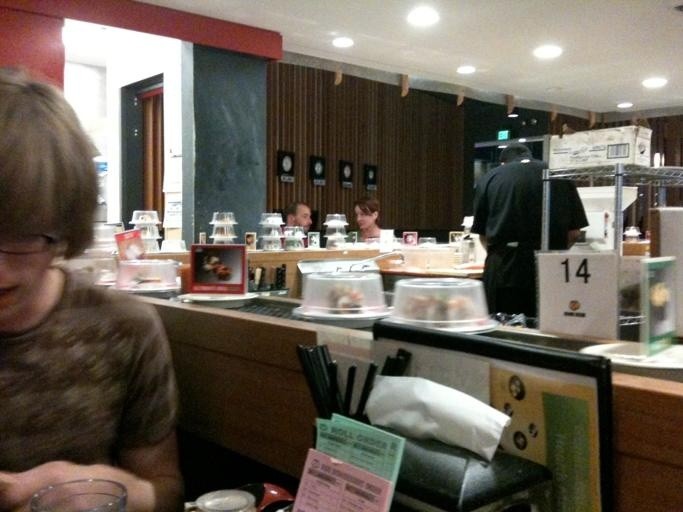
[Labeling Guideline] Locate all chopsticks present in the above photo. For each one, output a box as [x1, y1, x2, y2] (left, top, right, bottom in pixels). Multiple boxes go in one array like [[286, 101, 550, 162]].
[[293, 342, 412, 422]]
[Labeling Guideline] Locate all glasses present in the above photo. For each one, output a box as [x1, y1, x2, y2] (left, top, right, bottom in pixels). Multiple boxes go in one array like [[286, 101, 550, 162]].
[[0, 232, 62, 256]]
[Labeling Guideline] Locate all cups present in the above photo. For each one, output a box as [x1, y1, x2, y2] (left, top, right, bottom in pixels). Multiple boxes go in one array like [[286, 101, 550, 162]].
[[28, 480, 129, 510], [399, 245, 466, 273]]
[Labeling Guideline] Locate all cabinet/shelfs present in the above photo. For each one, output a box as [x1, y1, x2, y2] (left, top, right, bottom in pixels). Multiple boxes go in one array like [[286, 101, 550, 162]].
[[541, 160, 683, 326]]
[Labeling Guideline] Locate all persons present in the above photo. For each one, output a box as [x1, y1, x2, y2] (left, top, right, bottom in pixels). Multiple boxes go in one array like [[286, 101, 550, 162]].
[[351, 196, 394, 240], [472, 143, 588, 323], [280, 202, 314, 232], [0, 70, 186, 509]]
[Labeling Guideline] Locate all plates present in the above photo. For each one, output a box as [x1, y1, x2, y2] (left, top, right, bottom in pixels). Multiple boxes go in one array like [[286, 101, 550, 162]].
[[293, 307, 504, 338], [94, 281, 259, 310], [83, 218, 350, 251]]
